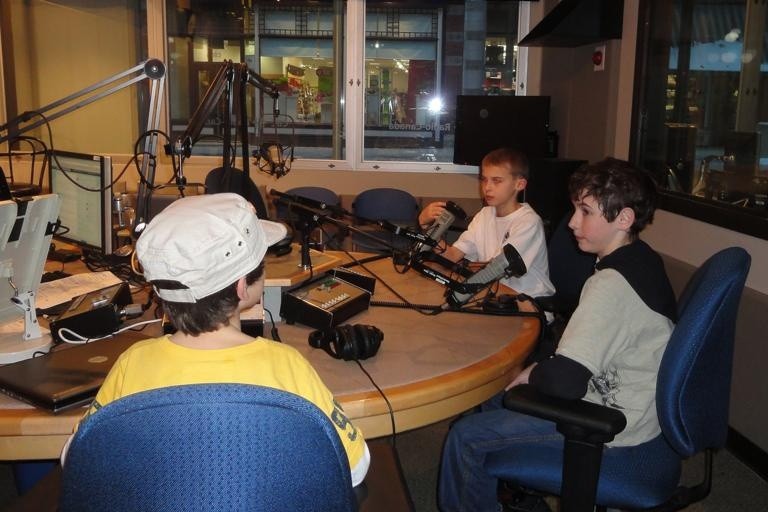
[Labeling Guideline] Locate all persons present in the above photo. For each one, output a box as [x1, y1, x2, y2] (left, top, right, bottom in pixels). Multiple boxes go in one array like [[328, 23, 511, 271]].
[[435, 157, 681, 509], [418, 149, 557, 327], [60, 192, 372, 488]]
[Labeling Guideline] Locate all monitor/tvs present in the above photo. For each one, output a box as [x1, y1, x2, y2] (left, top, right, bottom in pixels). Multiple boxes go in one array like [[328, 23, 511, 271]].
[[48, 150, 112, 254], [1, 194, 63, 364]]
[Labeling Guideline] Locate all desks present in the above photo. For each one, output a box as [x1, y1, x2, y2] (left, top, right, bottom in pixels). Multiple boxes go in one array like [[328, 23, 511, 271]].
[[2, 246, 540, 463]]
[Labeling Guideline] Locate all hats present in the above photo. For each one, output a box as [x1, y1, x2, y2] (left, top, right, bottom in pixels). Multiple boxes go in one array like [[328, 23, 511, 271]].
[[136, 194, 288, 302]]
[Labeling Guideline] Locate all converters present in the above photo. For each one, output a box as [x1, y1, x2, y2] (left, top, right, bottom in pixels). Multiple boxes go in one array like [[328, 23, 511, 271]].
[[50, 304, 117, 346], [48, 248, 81, 262]]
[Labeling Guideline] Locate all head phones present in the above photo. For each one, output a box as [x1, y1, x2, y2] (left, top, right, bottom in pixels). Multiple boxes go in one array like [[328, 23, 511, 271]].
[[308, 323, 384, 361]]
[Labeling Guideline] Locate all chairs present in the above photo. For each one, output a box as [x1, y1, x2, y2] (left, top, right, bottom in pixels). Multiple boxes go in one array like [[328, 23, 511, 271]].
[[351, 188, 420, 221], [486, 245, 753, 509], [283, 187, 340, 206], [50, 381, 358, 509]]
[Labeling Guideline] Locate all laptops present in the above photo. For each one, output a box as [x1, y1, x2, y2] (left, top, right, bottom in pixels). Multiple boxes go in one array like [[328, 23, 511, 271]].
[[0, 331, 159, 412]]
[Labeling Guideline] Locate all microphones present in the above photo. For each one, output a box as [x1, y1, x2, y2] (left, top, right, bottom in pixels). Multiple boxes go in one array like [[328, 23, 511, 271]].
[[430, 243, 527, 314], [404, 200, 467, 272]]
